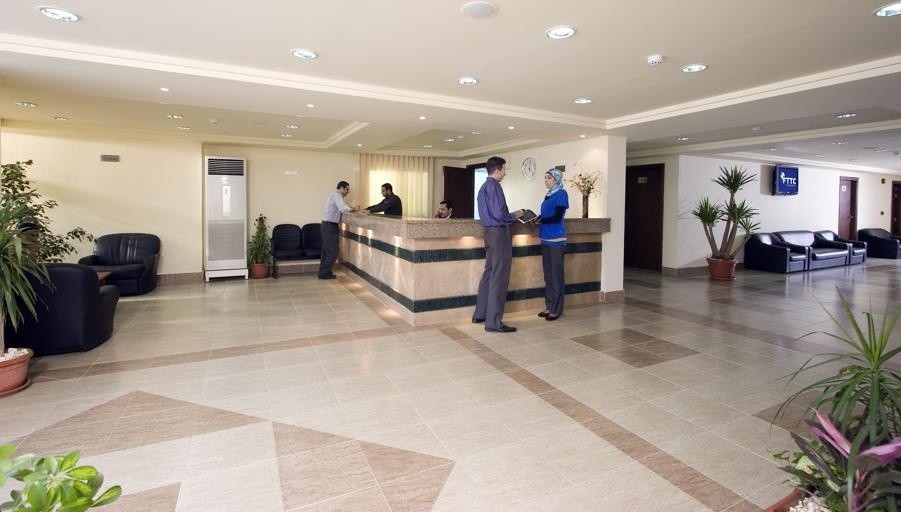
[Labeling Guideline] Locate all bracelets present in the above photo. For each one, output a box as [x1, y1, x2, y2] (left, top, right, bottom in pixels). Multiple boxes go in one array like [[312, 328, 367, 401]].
[[353, 207, 356, 212]]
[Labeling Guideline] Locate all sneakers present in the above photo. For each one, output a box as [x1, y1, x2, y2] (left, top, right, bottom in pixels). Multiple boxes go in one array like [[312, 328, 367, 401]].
[[318, 272, 335, 279]]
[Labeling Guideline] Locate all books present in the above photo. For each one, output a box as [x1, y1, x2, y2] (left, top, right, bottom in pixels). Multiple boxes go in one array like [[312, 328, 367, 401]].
[[517, 208, 537, 225]]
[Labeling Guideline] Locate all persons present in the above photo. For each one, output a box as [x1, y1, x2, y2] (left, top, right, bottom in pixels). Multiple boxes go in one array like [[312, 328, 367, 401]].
[[434, 200, 456, 219], [470, 154, 525, 335], [532, 166, 570, 323], [360, 181, 404, 218], [14, 216, 43, 262], [316, 180, 361, 280]]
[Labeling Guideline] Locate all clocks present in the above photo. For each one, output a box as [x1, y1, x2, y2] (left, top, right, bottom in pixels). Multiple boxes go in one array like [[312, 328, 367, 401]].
[[519, 156, 540, 181]]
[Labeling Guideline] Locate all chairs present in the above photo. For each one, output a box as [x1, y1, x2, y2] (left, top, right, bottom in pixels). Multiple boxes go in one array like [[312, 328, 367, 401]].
[[858, 226, 900, 258], [268, 222, 305, 279], [77, 233, 163, 297], [5, 261, 120, 356], [300, 222, 322, 260], [743, 230, 867, 273]]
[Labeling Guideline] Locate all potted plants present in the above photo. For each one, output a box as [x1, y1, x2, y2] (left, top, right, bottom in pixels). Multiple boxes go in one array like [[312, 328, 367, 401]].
[[754, 280, 899, 512], [0, 159, 97, 399], [249, 211, 273, 280], [689, 163, 762, 285]]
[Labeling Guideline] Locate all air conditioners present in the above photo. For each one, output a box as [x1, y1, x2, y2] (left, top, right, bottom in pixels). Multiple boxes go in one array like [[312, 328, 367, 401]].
[[201, 155, 248, 284]]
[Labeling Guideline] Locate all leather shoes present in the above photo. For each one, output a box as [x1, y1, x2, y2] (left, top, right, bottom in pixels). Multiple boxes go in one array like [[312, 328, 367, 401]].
[[538, 309, 560, 321], [485, 325, 516, 333]]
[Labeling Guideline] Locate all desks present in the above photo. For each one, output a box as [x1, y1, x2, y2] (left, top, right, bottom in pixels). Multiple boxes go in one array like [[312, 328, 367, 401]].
[[96, 270, 112, 288]]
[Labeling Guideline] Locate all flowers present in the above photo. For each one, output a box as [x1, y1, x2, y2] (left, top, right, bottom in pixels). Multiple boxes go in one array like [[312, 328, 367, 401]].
[[571, 173, 600, 192]]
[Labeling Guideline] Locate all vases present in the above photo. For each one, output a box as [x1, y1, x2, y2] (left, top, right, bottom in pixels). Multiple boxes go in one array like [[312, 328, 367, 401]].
[[581, 192, 589, 219]]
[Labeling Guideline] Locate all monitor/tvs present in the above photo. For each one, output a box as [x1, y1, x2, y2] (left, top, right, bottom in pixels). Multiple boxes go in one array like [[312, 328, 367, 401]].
[[772, 166, 799, 197]]
